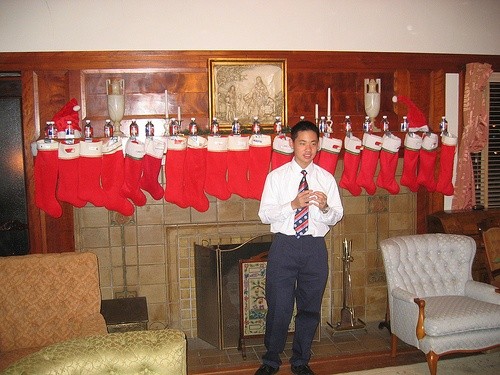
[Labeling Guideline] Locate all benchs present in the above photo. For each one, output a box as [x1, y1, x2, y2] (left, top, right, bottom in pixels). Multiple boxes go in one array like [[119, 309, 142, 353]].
[[0, 251, 187, 375]]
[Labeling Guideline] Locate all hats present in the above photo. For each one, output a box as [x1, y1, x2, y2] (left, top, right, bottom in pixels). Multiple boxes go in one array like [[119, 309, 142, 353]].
[[45, 99, 83, 139], [393, 96, 429, 132]]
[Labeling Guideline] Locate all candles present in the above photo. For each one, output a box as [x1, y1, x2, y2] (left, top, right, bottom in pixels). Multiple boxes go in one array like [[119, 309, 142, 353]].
[[164, 90, 169, 118], [178, 106, 181, 120], [315, 103, 318, 118], [327, 88, 331, 117]]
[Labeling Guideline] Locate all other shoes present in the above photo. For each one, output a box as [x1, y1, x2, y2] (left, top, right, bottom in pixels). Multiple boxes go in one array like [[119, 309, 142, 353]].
[[291, 364, 314, 375], [254, 363, 279, 375]]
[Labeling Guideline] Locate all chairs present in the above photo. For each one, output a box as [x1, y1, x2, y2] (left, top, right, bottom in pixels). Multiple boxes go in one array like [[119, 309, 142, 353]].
[[379, 233, 500, 375], [477, 216, 500, 291]]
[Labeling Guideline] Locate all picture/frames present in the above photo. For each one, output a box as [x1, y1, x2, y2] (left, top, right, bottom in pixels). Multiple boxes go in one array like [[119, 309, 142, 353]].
[[208, 58, 288, 131], [239, 252, 297, 338]]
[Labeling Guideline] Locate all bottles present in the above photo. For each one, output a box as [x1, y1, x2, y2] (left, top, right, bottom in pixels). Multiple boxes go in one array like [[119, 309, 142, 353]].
[[298, 116, 305, 121], [272, 116, 282, 134], [439, 117, 448, 136], [319, 116, 328, 133], [400, 116, 410, 132], [231, 117, 242, 135], [188, 118, 199, 135], [381, 115, 389, 133], [129, 118, 139, 137], [168, 117, 179, 136], [44, 121, 58, 139], [65, 121, 74, 138], [103, 119, 113, 137], [210, 117, 219, 134], [251, 116, 262, 135], [362, 116, 372, 133], [343, 116, 353, 132], [83, 120, 94, 139], [145, 120, 155, 137]]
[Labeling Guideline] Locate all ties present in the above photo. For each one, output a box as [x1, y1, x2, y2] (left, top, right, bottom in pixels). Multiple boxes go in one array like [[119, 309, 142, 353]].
[[294, 170, 308, 239]]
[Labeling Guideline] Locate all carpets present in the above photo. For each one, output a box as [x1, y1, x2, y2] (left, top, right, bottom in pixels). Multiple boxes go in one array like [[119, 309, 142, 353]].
[[335, 351, 500, 375]]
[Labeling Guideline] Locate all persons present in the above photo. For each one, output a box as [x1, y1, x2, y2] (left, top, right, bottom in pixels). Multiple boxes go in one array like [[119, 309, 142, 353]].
[[254, 120, 344, 375]]
